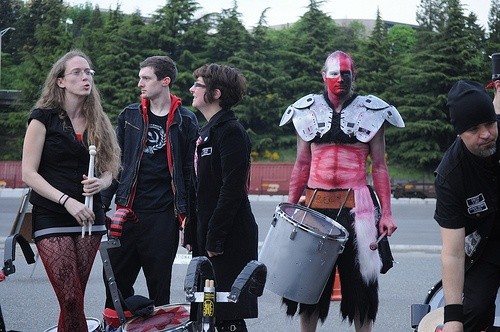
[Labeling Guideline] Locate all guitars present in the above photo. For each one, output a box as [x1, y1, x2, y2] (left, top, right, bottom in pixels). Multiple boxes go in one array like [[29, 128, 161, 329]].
[[257, 201, 349, 304]]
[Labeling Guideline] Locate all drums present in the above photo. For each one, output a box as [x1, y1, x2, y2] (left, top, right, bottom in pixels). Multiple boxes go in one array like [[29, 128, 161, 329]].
[[123, 302, 191, 332], [42, 317, 103, 332]]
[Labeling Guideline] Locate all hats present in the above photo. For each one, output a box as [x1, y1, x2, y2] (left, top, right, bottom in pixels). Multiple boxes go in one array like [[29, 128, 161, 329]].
[[491, 53, 500, 82], [449, 79, 498, 135]]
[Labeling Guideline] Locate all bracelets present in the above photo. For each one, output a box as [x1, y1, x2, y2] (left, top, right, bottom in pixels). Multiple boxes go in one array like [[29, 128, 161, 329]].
[[59, 193, 70, 206], [444, 304, 464, 323]]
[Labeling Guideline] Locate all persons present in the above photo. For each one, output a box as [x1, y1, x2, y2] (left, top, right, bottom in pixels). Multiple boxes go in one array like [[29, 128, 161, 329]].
[[182, 63, 259, 332], [279, 50, 405, 332], [21, 51, 121, 332], [101, 56, 198, 328], [433, 52, 500, 332]]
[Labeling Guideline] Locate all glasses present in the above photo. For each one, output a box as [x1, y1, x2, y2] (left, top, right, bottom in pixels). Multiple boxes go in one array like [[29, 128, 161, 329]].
[[64, 69, 95, 76], [194, 82, 206, 87]]
